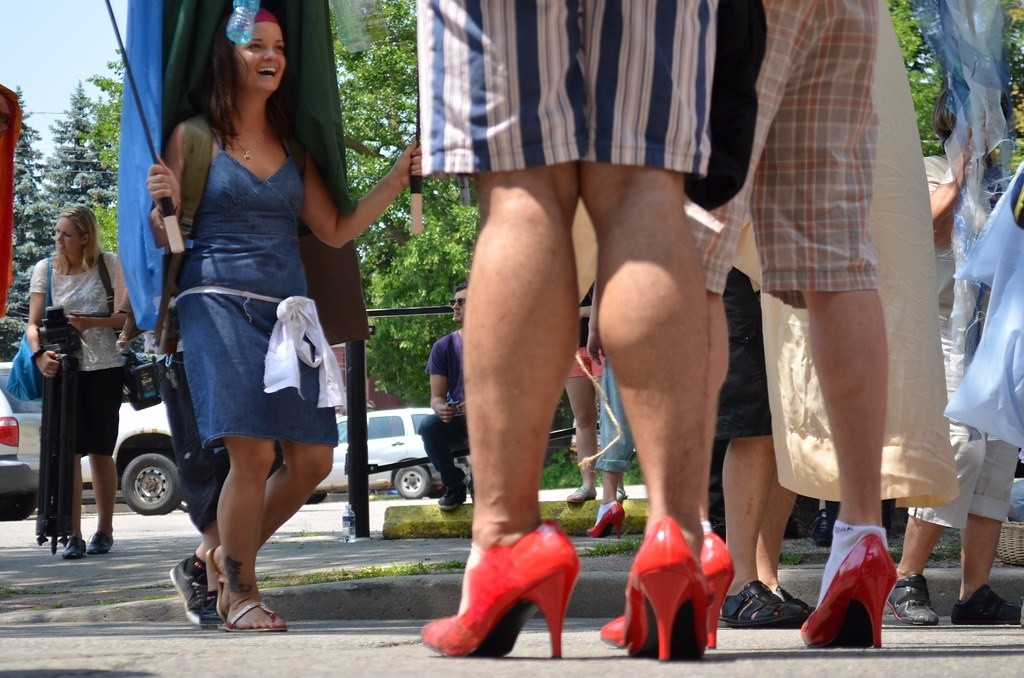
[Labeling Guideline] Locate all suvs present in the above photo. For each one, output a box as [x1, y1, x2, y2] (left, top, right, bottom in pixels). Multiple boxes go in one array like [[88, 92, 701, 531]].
[[0, 361, 43, 521]]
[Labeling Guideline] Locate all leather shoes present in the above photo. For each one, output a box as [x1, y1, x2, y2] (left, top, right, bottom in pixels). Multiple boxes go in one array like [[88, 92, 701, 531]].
[[952, 585, 1020, 624], [63, 537, 86, 559], [888, 574, 940, 626], [87, 532, 114, 555]]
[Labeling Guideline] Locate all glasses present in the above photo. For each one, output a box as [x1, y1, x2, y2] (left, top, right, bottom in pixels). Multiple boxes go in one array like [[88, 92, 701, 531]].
[[450, 297, 466, 306]]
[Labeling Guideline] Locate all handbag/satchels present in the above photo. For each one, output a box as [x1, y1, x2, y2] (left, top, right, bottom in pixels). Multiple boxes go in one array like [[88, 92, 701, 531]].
[[5, 257, 53, 402]]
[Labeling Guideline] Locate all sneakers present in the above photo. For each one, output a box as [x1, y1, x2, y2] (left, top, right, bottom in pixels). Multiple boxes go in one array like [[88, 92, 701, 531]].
[[169, 558, 224, 626], [808, 508, 832, 544], [438, 484, 474, 509]]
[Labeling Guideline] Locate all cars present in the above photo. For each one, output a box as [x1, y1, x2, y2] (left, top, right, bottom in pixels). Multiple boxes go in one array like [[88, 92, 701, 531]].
[[304, 407, 441, 505], [82, 386, 190, 516]]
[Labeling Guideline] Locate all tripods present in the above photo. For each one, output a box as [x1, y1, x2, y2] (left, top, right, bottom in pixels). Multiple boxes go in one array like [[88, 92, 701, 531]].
[[36, 305, 80, 554]]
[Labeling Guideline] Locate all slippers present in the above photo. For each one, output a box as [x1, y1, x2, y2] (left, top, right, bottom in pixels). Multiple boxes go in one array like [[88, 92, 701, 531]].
[[218, 601, 289, 632], [206, 546, 229, 623]]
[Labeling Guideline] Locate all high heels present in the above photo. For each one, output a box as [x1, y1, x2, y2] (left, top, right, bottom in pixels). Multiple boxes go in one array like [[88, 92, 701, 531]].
[[586, 502, 625, 539], [624, 519, 708, 663], [800, 533, 896, 648], [421, 520, 580, 659], [601, 531, 735, 649]]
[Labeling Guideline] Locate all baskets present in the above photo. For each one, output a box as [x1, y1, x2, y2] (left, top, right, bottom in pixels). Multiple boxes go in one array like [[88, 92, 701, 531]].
[[996, 516, 1024, 567]]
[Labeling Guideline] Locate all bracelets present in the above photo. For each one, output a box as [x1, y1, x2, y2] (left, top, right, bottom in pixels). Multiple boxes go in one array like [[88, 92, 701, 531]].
[[31, 347, 47, 363], [154, 203, 179, 224]]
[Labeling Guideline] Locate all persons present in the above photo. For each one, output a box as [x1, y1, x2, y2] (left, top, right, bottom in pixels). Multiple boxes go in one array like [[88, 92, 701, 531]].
[[420, 0, 1024, 664], [26, 205, 133, 559], [147, 0, 423, 634]]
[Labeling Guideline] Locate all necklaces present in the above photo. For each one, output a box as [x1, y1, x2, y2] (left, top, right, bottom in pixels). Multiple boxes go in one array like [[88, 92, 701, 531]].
[[232, 124, 267, 161]]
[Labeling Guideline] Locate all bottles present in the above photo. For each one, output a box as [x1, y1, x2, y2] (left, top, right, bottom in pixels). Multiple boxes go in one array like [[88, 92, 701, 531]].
[[343, 504, 355, 544]]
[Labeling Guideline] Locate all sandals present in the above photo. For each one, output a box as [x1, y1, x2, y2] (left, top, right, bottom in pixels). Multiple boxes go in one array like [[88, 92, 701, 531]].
[[567, 484, 595, 504], [616, 488, 627, 502], [718, 580, 816, 627]]
